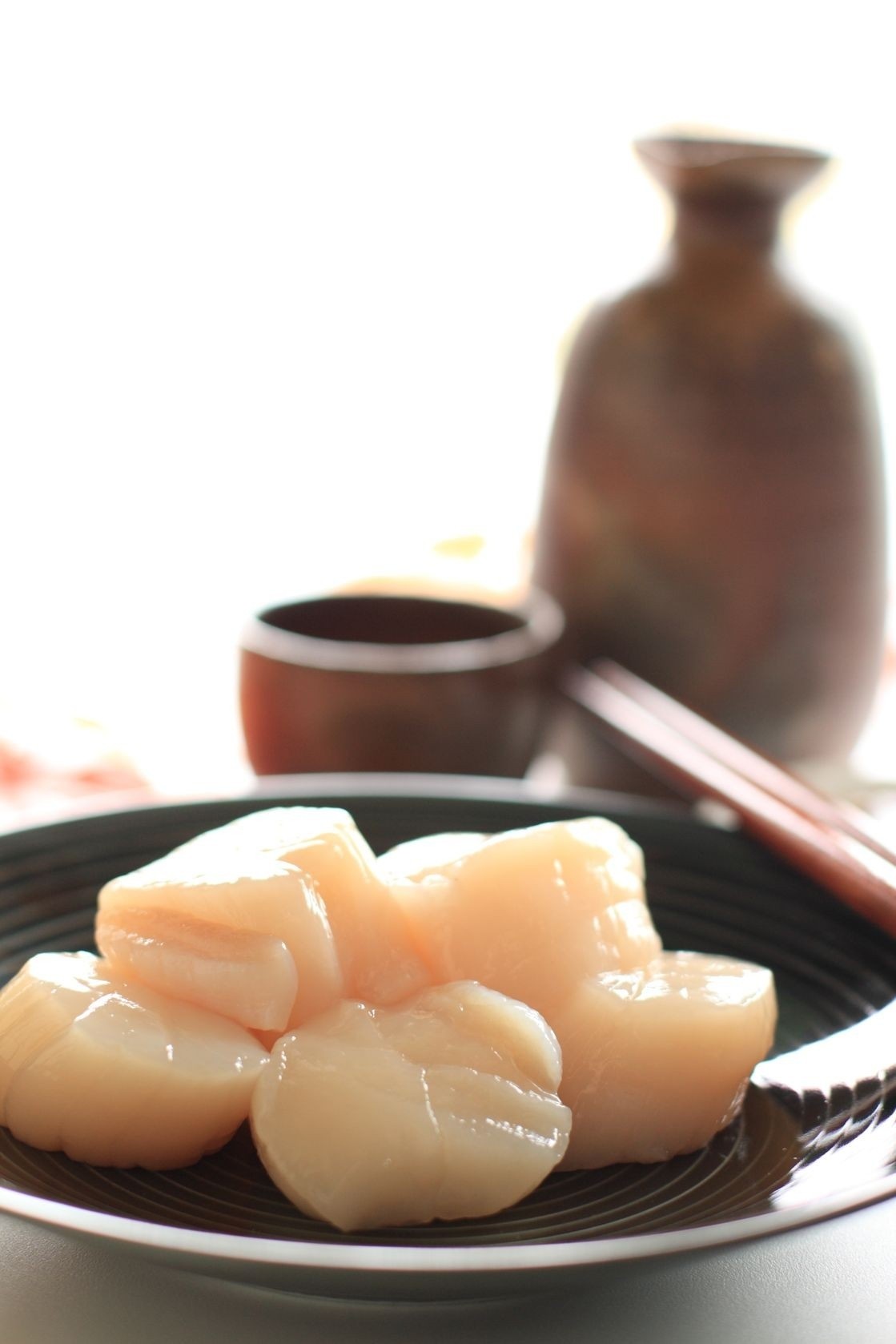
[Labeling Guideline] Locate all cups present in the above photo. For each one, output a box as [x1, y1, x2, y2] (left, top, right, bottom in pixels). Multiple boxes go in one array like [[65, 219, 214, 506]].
[[232, 588, 565, 779]]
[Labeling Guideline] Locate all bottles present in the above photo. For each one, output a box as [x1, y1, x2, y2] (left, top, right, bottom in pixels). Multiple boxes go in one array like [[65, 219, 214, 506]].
[[530, 131, 889, 805]]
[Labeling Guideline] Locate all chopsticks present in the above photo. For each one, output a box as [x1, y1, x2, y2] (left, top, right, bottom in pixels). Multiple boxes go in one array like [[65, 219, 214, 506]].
[[538, 653, 896, 928]]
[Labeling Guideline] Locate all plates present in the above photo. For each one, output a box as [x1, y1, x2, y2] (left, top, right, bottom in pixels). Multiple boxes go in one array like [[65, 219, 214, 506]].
[[0, 772, 896, 1307]]
